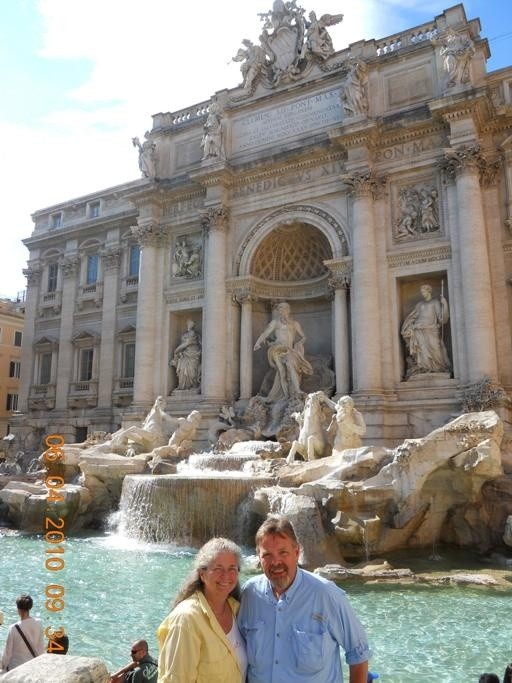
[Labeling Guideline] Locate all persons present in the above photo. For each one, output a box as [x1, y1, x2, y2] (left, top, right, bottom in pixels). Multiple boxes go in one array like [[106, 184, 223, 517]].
[[103, 392, 167, 445], [259, 0, 305, 66], [253, 301, 310, 396], [149, 405, 203, 462], [398, 282, 450, 378], [108, 637, 158, 682], [155, 536, 249, 682], [394, 211, 418, 240], [434, 26, 475, 88], [284, 388, 326, 461], [184, 247, 202, 279], [324, 394, 367, 454], [231, 38, 265, 90], [236, 516, 373, 682], [298, 10, 332, 59], [197, 102, 224, 161], [132, 129, 158, 178], [337, 53, 368, 114], [176, 238, 196, 273], [1, 593, 45, 673], [417, 187, 438, 232], [215, 394, 273, 445], [169, 318, 203, 393]]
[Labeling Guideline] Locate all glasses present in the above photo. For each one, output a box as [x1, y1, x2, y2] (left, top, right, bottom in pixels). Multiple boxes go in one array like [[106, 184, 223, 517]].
[[131, 649, 144, 655]]
